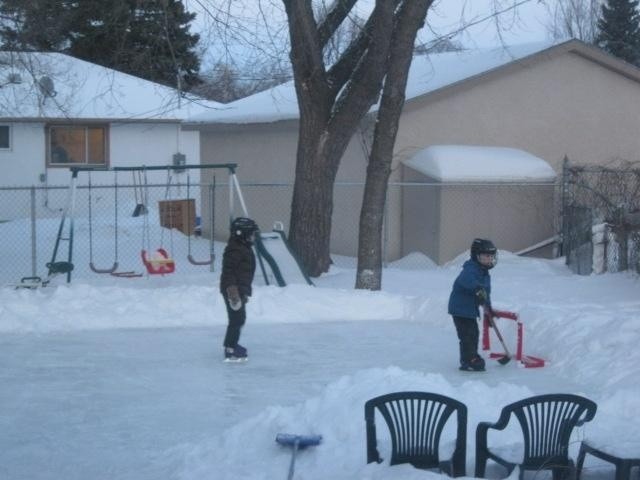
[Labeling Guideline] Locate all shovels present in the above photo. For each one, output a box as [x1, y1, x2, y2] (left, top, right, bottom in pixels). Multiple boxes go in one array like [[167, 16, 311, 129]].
[[481, 303, 525, 366]]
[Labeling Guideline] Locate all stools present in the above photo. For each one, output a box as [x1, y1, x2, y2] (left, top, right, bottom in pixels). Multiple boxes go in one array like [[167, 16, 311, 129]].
[[576, 440, 640, 480]]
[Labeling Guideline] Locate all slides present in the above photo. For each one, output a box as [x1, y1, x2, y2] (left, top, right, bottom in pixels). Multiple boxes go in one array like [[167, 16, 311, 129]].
[[259, 232, 311, 285]]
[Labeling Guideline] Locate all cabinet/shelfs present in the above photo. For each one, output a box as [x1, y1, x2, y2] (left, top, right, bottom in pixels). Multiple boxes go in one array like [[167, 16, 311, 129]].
[[159, 199, 196, 235]]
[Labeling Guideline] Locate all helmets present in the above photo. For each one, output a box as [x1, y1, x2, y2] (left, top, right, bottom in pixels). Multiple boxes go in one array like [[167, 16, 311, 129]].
[[231, 218, 259, 246], [471, 238, 497, 269]]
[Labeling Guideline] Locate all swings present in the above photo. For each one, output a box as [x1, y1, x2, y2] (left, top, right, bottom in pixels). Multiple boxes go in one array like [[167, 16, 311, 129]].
[[87, 171, 217, 279]]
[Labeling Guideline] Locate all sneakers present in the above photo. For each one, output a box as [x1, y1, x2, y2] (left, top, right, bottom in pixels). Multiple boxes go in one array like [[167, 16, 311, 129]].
[[461, 354, 484, 369], [225, 344, 248, 358]]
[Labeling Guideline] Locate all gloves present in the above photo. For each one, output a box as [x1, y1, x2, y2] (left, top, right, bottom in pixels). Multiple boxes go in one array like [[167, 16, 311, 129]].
[[226, 285, 242, 310], [485, 310, 500, 326], [473, 286, 488, 304]]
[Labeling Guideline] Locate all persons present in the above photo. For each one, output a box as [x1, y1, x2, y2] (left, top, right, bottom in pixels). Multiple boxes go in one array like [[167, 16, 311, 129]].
[[219, 217, 260, 358], [447, 238, 497, 371]]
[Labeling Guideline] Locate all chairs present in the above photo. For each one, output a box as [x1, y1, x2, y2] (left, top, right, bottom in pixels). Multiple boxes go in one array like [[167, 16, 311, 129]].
[[474, 393, 598, 480], [365, 392, 467, 478]]
